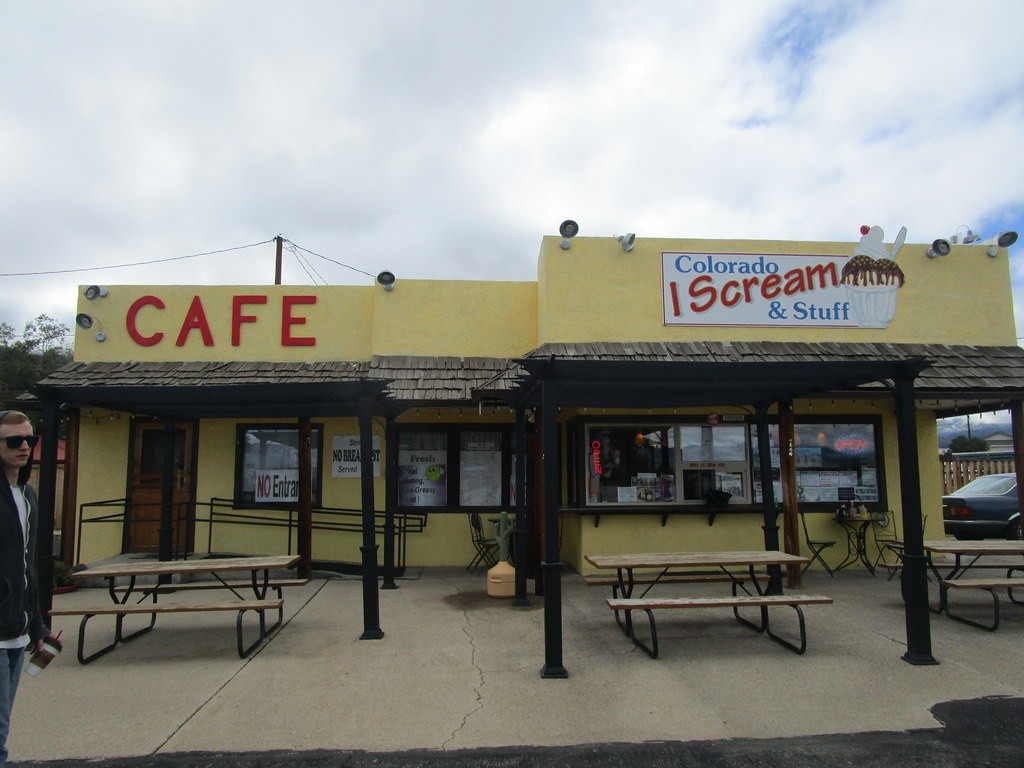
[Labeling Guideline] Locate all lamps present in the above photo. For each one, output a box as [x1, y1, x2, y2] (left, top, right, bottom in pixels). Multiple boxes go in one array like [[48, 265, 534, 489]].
[[560, 219, 579, 250], [378, 270, 396, 292], [952, 225, 982, 245], [612, 232, 636, 252], [76, 313, 106, 341], [988, 230, 1018, 257], [83, 285, 109, 300], [926, 238, 953, 259]]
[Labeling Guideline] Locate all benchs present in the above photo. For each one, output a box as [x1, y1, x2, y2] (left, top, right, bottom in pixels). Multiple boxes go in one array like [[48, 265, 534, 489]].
[[112, 578, 308, 638], [879, 563, 1024, 615], [940, 579, 1024, 631], [48, 599, 284, 666], [606, 594, 834, 659], [584, 573, 772, 637]]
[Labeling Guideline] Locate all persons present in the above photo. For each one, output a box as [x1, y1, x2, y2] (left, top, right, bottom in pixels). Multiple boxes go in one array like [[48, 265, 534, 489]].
[[0, 409, 61, 768]]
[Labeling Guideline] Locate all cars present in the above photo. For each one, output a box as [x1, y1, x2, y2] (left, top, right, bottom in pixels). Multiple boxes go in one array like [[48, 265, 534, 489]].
[[942, 473, 1021, 541]]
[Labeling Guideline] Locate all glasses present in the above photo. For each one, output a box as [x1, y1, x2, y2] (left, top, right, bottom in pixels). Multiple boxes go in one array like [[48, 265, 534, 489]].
[[0, 435, 40, 448]]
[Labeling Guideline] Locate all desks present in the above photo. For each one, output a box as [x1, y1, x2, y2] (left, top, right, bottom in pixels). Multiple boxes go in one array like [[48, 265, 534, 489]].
[[72, 555, 303, 641], [892, 539, 1024, 613], [584, 550, 811, 639], [832, 517, 886, 578], [489, 518, 517, 564]]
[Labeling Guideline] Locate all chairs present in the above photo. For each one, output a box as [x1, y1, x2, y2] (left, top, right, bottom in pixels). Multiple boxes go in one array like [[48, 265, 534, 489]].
[[466, 512, 501, 577], [799, 511, 836, 576], [870, 510, 932, 582]]
